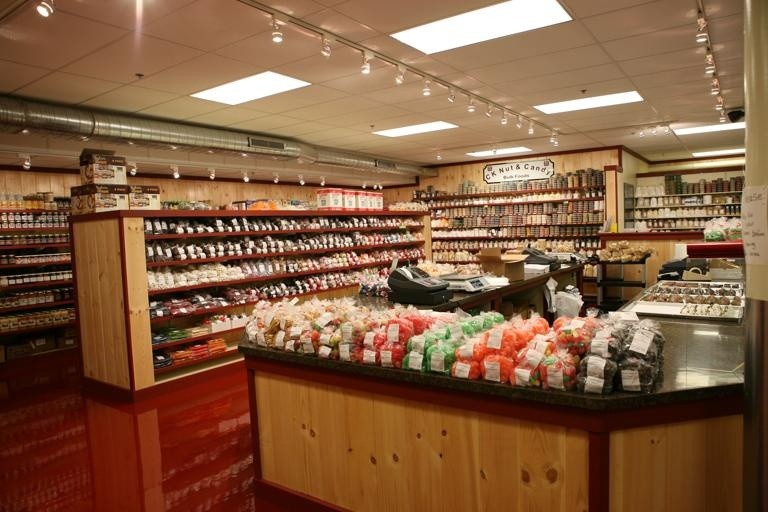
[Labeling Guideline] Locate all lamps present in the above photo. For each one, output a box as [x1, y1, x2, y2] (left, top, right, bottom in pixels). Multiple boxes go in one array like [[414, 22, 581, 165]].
[[33, 1, 61, 20], [14, 148, 385, 194], [686, 1, 732, 126], [260, 14, 573, 150]]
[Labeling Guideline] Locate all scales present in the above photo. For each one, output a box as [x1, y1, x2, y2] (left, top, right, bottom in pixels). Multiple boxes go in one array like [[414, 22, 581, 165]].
[[439, 273, 488, 293]]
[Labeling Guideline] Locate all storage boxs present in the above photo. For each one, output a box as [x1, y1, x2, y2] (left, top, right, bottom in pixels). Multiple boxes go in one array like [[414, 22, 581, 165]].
[[477, 245, 532, 283]]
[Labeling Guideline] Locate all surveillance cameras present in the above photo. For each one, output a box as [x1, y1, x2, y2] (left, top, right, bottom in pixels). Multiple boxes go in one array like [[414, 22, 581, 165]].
[[727, 108, 745, 122]]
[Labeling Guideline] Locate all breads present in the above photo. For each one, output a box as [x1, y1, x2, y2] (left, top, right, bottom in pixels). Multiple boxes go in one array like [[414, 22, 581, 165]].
[[597, 240, 654, 263]]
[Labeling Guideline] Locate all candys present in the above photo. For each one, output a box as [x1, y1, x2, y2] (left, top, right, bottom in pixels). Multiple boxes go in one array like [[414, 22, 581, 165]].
[[248, 299, 664, 394]]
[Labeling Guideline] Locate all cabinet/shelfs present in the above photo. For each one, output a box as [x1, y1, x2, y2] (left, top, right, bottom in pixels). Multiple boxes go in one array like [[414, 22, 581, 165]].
[[68, 182, 163, 216], [407, 183, 610, 265], [622, 183, 742, 233], [1, 189, 79, 365], [141, 205, 434, 383]]
